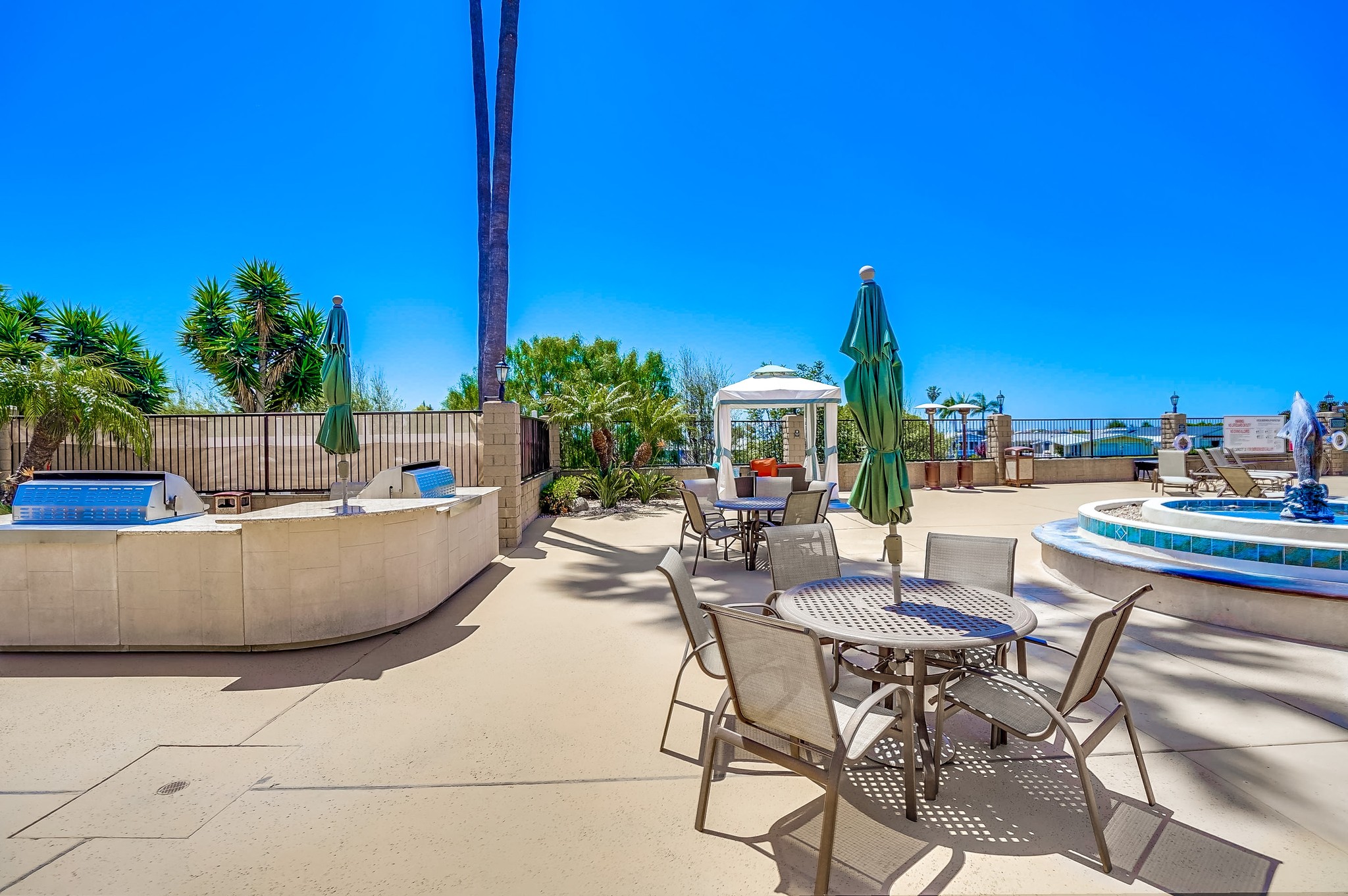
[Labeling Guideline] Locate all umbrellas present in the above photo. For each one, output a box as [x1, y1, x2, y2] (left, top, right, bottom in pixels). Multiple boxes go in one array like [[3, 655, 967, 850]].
[[317, 305, 361, 515], [839, 279, 915, 605]]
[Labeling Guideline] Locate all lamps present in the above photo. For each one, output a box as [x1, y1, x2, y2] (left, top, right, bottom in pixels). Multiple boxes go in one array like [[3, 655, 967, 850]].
[[1170, 391, 1180, 414], [544, 391, 553, 413], [1324, 391, 1336, 412], [494, 353, 511, 402], [996, 390, 1005, 415]]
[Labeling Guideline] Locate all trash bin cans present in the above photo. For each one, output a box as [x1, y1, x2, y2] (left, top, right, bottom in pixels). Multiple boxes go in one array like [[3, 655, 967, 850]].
[[1004, 446, 1034, 487], [211, 492, 252, 514]]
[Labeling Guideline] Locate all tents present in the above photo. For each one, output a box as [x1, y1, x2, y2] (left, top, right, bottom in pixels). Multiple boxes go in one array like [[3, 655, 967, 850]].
[[712, 364, 842, 502]]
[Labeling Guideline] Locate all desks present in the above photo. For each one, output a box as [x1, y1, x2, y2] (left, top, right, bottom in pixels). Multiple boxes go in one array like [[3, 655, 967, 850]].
[[712, 495, 812, 572], [777, 572, 1040, 771]]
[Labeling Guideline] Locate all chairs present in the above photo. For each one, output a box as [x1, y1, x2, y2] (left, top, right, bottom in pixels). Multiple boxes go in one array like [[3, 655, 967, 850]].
[[681, 480, 734, 557], [656, 546, 817, 774], [882, 529, 1021, 753], [931, 580, 1158, 878], [1149, 449, 1197, 498], [750, 487, 827, 573], [1188, 446, 1298, 499], [746, 475, 795, 552], [787, 480, 840, 561], [760, 521, 869, 693], [695, 601, 920, 896], [674, 485, 750, 577], [746, 457, 776, 477], [771, 462, 807, 494], [705, 461, 755, 503]]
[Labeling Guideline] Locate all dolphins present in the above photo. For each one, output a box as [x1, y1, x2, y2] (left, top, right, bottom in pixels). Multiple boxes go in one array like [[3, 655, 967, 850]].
[[1275, 389, 1330, 511]]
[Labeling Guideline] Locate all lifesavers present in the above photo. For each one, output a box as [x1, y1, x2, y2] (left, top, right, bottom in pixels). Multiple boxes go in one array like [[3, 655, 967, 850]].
[[1330, 431, 1348, 450], [1172, 433, 1191, 454]]
